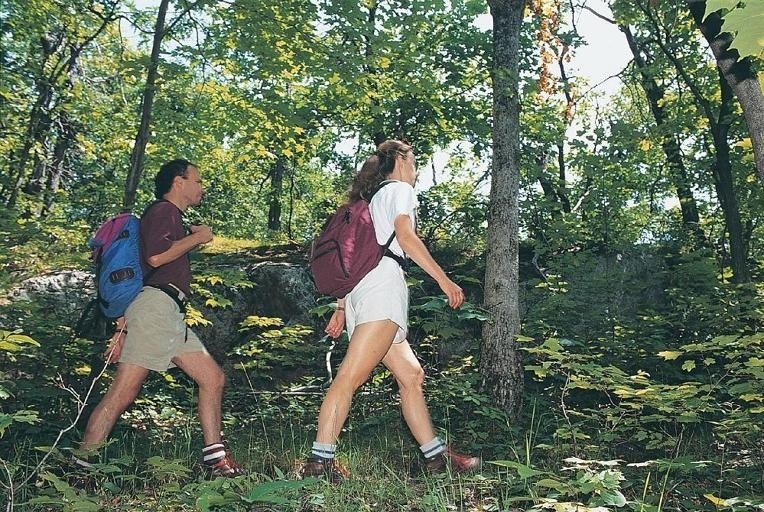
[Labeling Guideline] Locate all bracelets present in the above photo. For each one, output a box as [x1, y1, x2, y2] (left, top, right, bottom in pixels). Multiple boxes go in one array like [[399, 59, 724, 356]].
[[336, 307, 344, 311], [115, 329, 127, 335]]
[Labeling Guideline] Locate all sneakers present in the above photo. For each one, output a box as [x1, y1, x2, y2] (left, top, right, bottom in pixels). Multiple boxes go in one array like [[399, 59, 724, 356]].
[[62, 462, 98, 498], [424, 450, 480, 478], [203, 456, 248, 480], [303, 456, 344, 483]]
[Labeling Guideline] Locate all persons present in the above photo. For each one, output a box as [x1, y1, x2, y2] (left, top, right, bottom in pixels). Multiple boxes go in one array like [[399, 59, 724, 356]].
[[302, 138, 481, 484], [70, 158, 251, 491]]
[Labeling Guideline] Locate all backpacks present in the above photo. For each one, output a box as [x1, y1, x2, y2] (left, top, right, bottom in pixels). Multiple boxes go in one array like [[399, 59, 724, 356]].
[[312, 180, 407, 298], [88, 200, 186, 319]]
